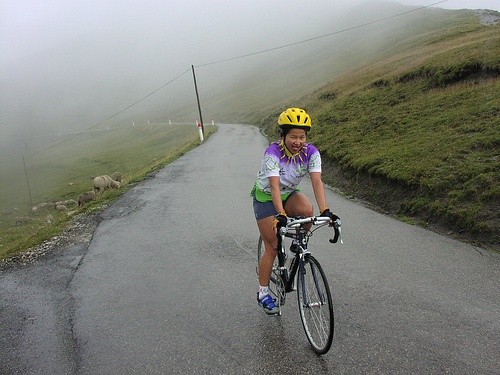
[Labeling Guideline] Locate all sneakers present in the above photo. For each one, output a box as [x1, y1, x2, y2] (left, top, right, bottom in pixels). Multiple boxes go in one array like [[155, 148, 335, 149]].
[[257, 292, 280, 315]]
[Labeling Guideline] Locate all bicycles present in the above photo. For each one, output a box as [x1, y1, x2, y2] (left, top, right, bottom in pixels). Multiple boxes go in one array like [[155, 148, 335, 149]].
[[255, 211, 343, 355]]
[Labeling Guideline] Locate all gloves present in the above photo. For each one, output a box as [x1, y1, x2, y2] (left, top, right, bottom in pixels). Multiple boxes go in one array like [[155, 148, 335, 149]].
[[272, 210, 287, 237], [320, 209, 341, 229]]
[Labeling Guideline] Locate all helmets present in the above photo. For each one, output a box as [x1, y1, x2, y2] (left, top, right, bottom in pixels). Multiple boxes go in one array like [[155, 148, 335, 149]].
[[277, 107, 312, 132]]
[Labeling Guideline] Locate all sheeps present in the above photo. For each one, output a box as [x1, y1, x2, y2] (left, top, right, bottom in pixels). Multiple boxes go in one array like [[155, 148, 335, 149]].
[[36, 172, 121, 208]]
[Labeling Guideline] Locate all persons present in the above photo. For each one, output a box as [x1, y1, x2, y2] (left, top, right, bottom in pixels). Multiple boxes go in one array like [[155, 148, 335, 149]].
[[252, 108, 338, 315]]
